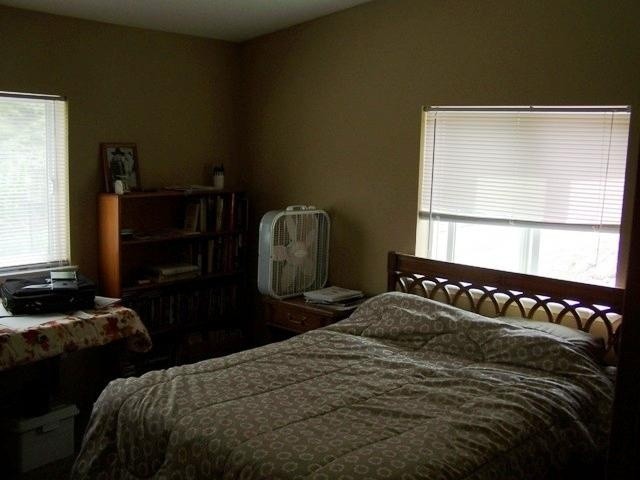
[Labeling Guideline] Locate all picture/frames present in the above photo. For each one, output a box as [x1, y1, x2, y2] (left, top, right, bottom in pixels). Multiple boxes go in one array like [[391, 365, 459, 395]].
[[100, 142, 142, 193]]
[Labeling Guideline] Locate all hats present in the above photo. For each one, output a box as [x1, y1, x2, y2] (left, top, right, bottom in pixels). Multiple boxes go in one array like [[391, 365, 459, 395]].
[[111, 148, 125, 156]]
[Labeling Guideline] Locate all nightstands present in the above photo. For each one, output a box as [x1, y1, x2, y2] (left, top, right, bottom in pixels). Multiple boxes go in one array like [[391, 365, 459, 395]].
[[262, 295, 370, 344]]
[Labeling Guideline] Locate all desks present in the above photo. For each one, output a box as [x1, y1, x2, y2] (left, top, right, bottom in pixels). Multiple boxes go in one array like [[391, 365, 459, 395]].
[[0, 296, 153, 371]]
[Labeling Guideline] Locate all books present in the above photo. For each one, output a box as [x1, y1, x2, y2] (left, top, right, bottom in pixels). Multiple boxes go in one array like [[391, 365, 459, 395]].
[[303, 286, 369, 311], [152, 183, 225, 283]]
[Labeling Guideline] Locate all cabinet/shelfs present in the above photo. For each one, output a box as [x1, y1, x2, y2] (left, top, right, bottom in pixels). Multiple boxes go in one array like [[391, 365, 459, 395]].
[[97, 185, 235, 369]]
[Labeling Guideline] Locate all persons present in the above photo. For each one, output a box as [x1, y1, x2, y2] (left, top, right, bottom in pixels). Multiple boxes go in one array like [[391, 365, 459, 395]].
[[109, 147, 137, 190]]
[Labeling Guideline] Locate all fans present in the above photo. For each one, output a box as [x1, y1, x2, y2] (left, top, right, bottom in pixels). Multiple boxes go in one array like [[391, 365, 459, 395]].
[[258, 204, 331, 300]]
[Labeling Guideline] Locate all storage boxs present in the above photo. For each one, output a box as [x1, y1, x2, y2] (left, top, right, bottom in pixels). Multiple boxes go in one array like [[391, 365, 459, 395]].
[[9, 403, 81, 473]]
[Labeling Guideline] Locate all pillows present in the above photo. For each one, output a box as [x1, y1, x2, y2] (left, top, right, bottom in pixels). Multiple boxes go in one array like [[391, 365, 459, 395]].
[[492, 316, 605, 368]]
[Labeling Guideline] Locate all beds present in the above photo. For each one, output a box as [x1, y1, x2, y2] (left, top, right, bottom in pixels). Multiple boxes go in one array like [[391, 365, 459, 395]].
[[70, 251, 624, 480]]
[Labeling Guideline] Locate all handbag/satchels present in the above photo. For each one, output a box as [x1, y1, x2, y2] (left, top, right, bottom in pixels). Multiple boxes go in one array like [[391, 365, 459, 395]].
[[1, 271, 95, 316]]
